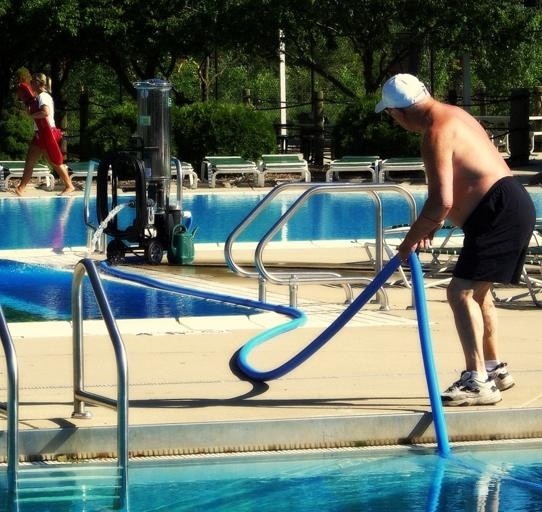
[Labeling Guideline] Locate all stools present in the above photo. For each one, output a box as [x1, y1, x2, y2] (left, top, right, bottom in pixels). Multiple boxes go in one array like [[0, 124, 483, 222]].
[[383, 215, 542, 312]]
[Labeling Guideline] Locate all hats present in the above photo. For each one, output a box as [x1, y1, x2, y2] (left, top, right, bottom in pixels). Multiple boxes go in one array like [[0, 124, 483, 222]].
[[374, 72, 429, 114]]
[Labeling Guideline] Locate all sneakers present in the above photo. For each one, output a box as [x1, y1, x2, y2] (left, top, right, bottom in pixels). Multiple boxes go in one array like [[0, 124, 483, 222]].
[[485, 361, 515, 393], [439, 369, 503, 408]]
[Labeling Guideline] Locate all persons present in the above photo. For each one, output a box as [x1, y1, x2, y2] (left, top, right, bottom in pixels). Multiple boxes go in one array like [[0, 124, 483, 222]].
[[5, 72, 75, 197], [373, 71, 537, 405]]
